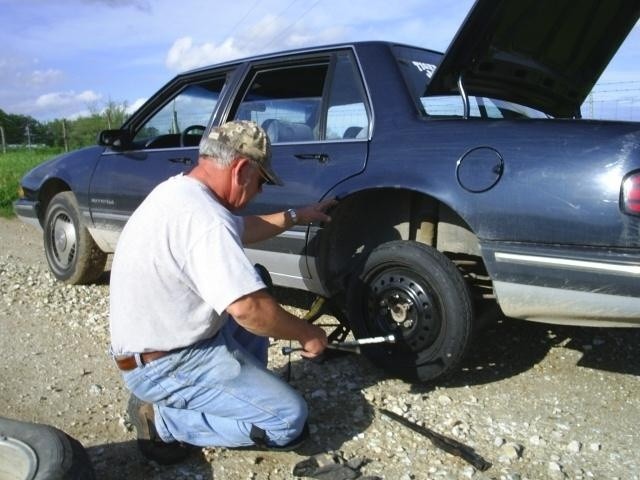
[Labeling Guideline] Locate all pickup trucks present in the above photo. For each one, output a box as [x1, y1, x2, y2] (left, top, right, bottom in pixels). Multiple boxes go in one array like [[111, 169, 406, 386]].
[[11, 1, 640, 385]]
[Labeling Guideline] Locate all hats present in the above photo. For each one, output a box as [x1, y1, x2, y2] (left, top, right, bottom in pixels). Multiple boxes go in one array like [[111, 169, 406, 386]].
[[210, 121, 285, 185]]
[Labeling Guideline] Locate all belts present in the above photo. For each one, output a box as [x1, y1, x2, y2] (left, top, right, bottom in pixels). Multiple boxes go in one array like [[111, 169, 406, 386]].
[[115, 349, 183, 372]]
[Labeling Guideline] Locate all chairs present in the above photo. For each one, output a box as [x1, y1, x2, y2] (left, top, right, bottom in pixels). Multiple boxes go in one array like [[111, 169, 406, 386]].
[[262, 118, 313, 144], [343, 126, 368, 138]]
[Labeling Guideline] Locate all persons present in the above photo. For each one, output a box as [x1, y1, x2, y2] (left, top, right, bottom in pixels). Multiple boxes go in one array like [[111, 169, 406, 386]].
[[106, 119, 342, 466]]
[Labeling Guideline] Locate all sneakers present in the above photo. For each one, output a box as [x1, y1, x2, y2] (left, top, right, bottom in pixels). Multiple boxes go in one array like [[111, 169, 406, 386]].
[[128, 393, 187, 462]]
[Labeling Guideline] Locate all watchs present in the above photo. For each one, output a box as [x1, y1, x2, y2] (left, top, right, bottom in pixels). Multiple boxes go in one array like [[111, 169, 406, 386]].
[[286, 207, 299, 225]]
